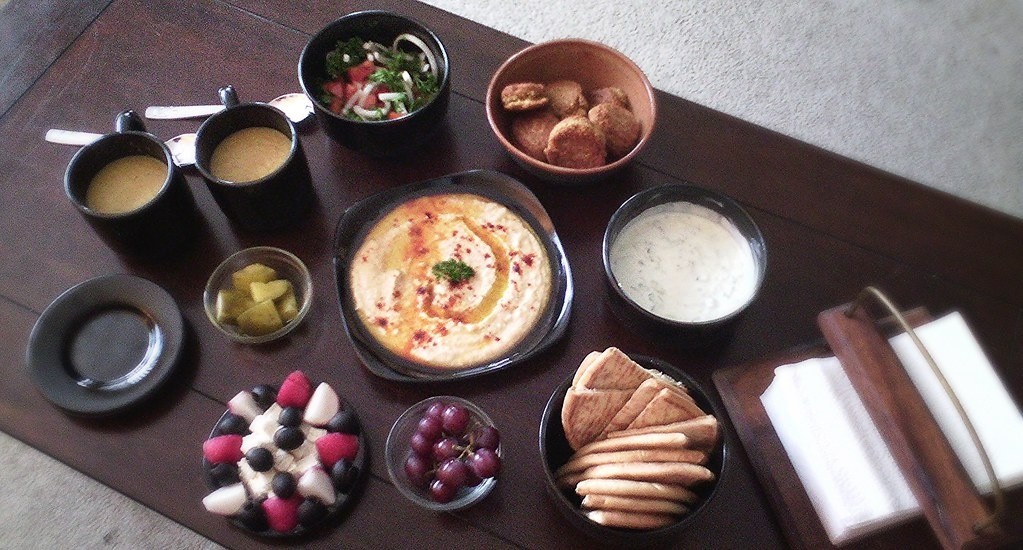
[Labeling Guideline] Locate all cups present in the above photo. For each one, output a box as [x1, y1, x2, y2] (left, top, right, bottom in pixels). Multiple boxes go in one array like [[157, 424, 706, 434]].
[[45, 128, 194, 254], [191, 84, 312, 224]]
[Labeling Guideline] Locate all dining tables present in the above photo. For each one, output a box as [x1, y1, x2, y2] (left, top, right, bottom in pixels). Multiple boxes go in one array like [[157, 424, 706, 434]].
[[0, 0, 1023, 550]]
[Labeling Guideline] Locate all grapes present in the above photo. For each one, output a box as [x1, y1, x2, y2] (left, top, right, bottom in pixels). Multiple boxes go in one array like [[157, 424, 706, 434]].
[[404, 400, 502, 504]]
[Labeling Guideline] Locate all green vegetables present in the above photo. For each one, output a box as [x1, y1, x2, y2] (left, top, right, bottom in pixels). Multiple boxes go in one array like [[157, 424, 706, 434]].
[[432, 258, 475, 284], [319, 35, 440, 121]]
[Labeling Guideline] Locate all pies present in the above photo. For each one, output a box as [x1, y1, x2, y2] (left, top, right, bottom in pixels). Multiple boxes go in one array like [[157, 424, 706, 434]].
[[555, 347, 718, 530]]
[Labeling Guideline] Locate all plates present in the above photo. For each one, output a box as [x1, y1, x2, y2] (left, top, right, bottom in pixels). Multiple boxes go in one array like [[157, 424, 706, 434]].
[[202, 381, 369, 539], [23, 271, 185, 417]]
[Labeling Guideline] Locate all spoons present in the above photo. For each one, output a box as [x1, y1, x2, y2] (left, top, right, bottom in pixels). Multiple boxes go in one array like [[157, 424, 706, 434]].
[[45, 129, 196, 167], [144, 92, 314, 124]]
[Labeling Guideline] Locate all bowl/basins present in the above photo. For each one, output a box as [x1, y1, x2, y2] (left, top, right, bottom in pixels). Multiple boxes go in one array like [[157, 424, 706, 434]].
[[537, 353, 728, 544], [600, 181, 769, 331], [331, 166, 575, 384], [202, 246, 314, 347], [484, 38, 658, 185], [297, 9, 454, 151], [384, 395, 504, 513]]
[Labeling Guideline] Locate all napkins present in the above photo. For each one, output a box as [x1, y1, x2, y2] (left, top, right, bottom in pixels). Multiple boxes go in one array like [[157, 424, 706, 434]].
[[761, 311, 1023, 544]]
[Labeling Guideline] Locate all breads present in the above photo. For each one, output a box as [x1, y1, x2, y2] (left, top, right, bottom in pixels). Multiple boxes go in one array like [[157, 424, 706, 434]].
[[501, 80, 639, 170]]
